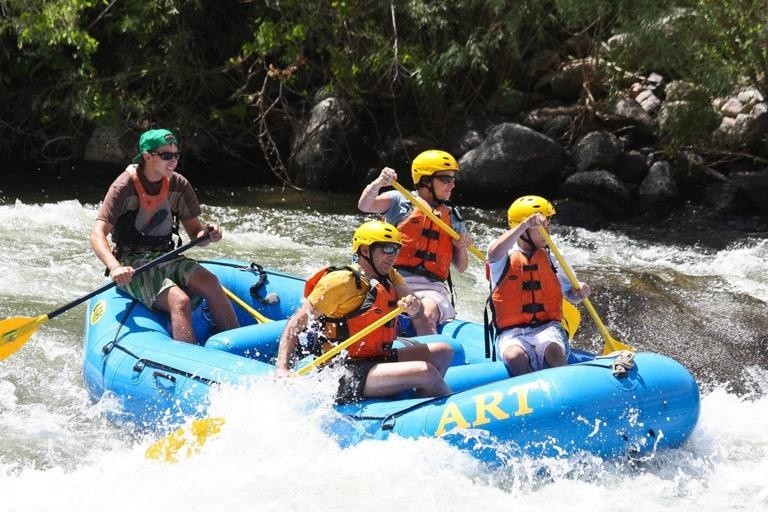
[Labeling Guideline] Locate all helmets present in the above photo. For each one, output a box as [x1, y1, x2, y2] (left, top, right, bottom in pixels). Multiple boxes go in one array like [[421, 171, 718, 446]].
[[507, 195, 557, 230], [353, 220, 402, 253], [411, 150, 459, 184]]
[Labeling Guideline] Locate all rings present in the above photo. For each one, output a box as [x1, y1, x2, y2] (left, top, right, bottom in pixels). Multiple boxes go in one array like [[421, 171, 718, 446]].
[[412, 306, 417, 310]]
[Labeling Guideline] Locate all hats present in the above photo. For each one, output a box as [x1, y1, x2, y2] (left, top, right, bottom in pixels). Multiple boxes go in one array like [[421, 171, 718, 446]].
[[133, 128, 178, 163]]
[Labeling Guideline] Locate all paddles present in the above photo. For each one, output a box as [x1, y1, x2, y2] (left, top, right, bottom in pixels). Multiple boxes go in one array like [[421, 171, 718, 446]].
[[145, 296, 409, 463], [537, 225, 633, 356], [0, 227, 213, 363]]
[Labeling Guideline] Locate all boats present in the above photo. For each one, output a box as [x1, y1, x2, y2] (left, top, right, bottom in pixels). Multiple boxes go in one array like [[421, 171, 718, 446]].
[[80, 263, 699, 474]]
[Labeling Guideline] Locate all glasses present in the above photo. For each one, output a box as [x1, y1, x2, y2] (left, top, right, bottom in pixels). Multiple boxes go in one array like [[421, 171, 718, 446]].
[[151, 151, 180, 160], [433, 175, 455, 184], [374, 244, 400, 255]]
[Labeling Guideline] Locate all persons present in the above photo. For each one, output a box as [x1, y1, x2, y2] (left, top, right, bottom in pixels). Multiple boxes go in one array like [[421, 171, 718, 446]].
[[484, 193, 593, 378], [87, 127, 244, 346], [359, 146, 477, 335], [272, 218, 454, 400]]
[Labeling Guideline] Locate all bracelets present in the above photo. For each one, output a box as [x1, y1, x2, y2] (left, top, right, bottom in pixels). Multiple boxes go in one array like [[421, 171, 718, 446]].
[[362, 184, 379, 200]]
